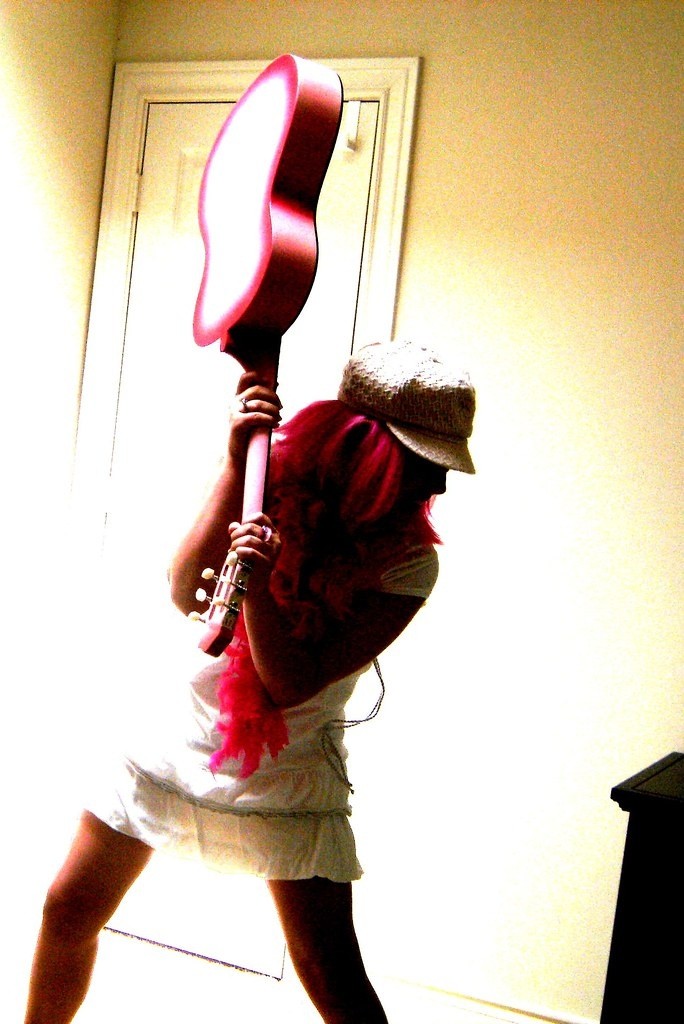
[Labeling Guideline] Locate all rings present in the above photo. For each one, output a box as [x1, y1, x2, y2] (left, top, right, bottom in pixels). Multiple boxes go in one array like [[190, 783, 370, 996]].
[[260, 524, 274, 542], [242, 398, 248, 411]]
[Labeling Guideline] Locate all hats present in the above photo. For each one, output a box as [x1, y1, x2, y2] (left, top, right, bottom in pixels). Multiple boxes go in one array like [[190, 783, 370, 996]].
[[339, 335, 477, 475]]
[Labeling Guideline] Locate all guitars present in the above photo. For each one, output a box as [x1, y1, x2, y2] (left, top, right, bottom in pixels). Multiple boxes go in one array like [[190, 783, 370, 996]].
[[181, 51, 345, 657]]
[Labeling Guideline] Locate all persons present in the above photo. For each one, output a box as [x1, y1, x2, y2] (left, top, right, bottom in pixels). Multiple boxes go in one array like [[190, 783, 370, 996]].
[[24, 342, 472, 1023]]
